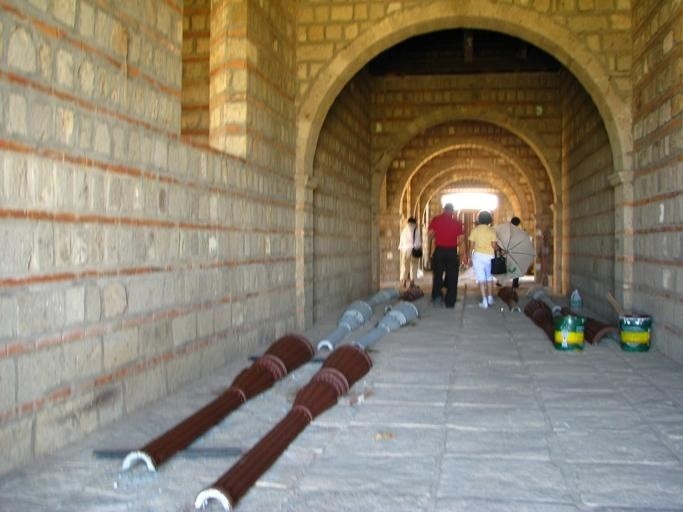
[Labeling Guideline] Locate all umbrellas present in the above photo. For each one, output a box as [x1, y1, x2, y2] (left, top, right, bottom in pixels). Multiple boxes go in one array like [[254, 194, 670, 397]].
[[493, 222, 536, 281]]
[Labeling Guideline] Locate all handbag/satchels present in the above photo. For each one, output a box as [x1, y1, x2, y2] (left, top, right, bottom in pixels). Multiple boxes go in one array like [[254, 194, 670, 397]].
[[491, 255, 507, 275], [411, 248, 422, 258]]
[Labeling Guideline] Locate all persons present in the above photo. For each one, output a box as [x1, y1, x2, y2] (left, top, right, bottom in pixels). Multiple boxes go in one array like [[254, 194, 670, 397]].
[[427, 201, 464, 307], [468, 210, 499, 308], [398, 216, 422, 288], [495, 217, 522, 288]]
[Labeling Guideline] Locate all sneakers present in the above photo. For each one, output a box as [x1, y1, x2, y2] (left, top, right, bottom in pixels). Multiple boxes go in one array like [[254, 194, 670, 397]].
[[478, 299, 496, 309], [435, 296, 454, 309]]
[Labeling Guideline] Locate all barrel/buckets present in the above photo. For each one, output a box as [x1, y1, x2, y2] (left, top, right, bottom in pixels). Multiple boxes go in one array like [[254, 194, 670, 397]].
[[617, 315, 653, 353], [554, 316, 588, 351], [571, 288, 583, 316]]
